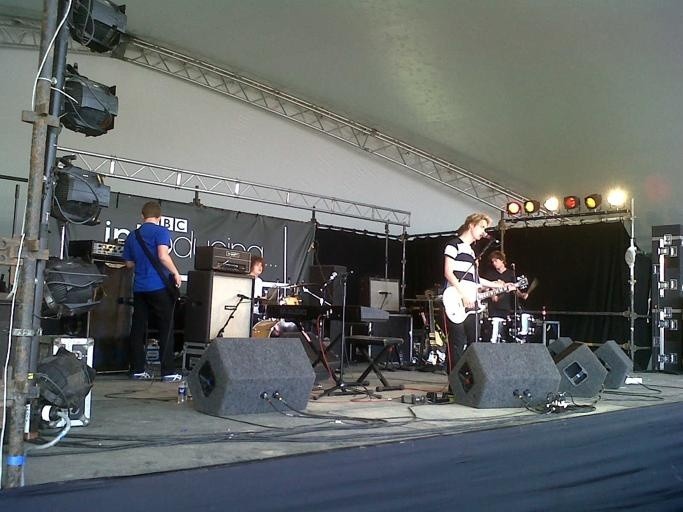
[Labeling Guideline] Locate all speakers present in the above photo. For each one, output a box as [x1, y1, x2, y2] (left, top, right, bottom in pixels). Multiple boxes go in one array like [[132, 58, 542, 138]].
[[186, 336, 317, 416], [64, 258, 135, 375], [359, 276, 401, 314], [555, 341, 608, 401], [447, 340, 563, 409], [182, 270, 256, 341], [595, 337, 633, 389], [549, 337, 571, 351]]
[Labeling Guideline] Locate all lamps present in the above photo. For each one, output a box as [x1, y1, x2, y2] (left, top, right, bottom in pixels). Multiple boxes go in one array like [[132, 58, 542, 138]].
[[71, 1, 130, 54], [43, 258, 107, 319], [51, 158, 112, 227], [37, 346, 97, 414], [507, 202, 520, 215], [584, 194, 602, 209], [564, 196, 580, 210], [524, 200, 540, 213], [66, 62, 119, 137]]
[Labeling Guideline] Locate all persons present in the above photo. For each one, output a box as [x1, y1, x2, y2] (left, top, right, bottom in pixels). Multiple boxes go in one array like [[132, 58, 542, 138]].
[[250, 256, 263, 327], [439, 212, 517, 398], [485, 250, 528, 343], [122, 201, 185, 381]]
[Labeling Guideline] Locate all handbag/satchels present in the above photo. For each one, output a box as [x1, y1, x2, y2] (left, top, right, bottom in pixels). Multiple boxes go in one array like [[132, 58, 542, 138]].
[[166, 273, 181, 302]]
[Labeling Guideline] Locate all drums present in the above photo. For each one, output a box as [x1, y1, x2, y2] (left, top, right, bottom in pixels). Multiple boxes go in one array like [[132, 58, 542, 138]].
[[508, 313, 536, 336], [479, 318, 513, 343], [252, 318, 298, 339]]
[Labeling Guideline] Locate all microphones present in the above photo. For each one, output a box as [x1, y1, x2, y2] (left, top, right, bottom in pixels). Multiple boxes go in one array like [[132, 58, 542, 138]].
[[482, 230, 492, 243], [379, 291, 393, 296], [326, 270, 340, 284]]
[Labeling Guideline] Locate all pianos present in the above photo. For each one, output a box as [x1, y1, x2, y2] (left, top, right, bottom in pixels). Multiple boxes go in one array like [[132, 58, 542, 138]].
[[265, 305, 389, 324]]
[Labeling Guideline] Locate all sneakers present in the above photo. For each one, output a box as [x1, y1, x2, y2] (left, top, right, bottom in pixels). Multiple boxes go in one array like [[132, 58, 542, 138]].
[[131, 369, 155, 380], [161, 372, 188, 383]]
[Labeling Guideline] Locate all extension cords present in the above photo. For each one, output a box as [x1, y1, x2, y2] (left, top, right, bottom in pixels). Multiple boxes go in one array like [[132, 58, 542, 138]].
[[626, 376, 642, 385]]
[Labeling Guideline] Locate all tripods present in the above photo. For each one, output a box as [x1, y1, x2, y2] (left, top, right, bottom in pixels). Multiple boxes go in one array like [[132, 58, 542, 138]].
[[313, 282, 383, 401]]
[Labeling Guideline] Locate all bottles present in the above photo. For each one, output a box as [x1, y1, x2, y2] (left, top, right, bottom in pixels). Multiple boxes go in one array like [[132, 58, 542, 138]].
[[176, 380, 186, 405]]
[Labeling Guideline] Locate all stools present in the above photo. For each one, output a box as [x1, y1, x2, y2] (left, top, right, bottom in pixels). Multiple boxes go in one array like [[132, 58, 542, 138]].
[[345, 335, 404, 391]]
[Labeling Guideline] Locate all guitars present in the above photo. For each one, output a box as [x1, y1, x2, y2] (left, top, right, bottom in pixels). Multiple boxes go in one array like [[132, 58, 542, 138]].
[[442, 275, 528, 324]]
